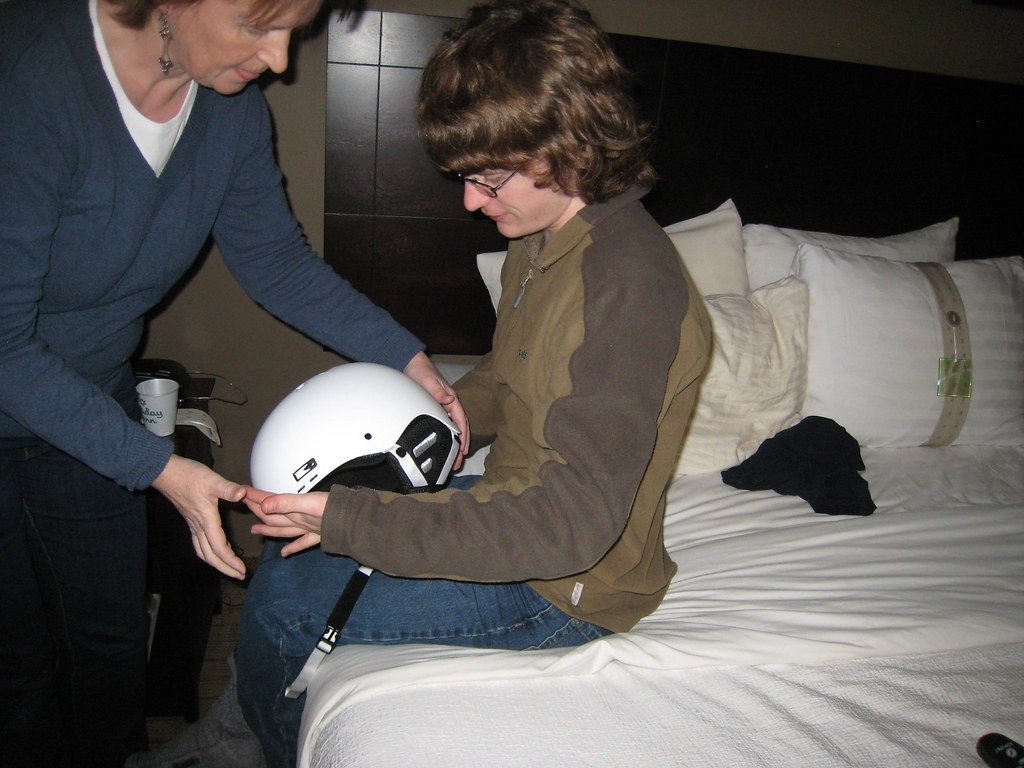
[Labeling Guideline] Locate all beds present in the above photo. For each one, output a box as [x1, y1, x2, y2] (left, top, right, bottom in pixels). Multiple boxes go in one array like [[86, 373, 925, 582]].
[[295, 9, 1023, 768]]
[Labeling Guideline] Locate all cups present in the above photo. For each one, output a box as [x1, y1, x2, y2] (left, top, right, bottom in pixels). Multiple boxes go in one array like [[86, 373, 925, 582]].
[[136, 379, 179, 437]]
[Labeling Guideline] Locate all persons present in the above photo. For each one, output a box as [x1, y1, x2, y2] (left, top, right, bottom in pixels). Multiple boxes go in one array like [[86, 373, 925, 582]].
[[234, 0, 714, 768], [1, 1, 468, 768]]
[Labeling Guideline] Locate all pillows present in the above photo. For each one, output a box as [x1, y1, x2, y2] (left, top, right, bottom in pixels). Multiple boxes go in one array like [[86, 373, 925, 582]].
[[789, 243, 1022, 450], [664, 276, 808, 482], [477, 197, 748, 320], [743, 217, 960, 292]]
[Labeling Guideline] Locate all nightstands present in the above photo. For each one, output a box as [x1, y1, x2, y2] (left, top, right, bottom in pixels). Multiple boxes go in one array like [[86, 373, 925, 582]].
[[143, 379, 223, 726]]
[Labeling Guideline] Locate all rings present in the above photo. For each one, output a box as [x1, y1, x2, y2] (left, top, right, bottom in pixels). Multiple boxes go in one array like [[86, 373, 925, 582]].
[[447, 413, 452, 418]]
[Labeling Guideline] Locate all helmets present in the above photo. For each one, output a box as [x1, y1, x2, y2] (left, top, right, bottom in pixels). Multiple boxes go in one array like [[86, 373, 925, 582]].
[[249, 363, 462, 494]]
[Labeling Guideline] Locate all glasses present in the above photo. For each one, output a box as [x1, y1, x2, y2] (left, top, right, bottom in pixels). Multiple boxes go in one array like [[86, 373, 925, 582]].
[[443, 156, 535, 198]]
